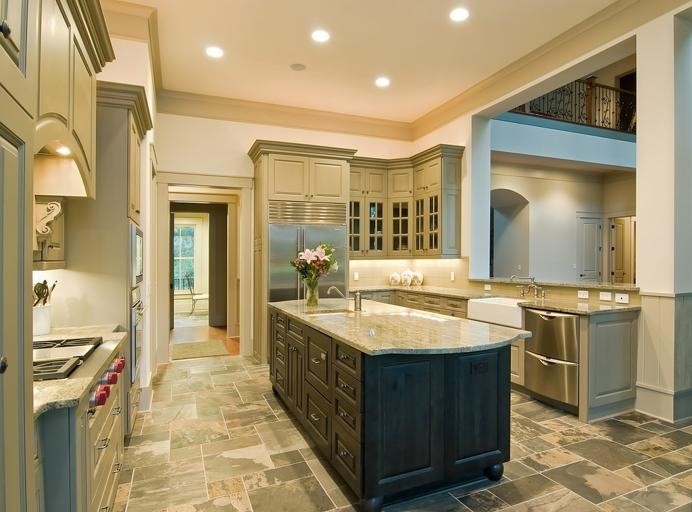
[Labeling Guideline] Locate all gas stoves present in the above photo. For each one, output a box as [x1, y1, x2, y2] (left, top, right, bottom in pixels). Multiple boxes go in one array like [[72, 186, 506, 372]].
[[33, 334, 102, 381]]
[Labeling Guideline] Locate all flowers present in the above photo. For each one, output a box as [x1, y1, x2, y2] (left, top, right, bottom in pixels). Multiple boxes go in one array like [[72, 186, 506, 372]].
[[290, 242, 339, 300]]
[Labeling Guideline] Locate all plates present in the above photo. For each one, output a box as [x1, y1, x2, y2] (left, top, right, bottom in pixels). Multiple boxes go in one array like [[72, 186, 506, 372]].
[[389, 269, 423, 287]]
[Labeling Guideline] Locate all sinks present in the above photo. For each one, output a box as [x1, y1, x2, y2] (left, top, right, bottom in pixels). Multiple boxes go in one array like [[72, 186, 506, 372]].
[[467, 297, 529, 328], [301, 308, 354, 315]]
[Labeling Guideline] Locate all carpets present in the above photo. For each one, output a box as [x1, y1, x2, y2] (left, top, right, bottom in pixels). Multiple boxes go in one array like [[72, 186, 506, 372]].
[[173, 339, 230, 360]]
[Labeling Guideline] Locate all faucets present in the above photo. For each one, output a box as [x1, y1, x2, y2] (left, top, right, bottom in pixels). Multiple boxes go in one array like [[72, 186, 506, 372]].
[[510, 275, 535, 284], [326, 286, 346, 302]]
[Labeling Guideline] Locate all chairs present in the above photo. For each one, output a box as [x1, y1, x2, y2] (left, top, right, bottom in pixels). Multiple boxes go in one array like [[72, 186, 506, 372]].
[[184, 272, 209, 316]]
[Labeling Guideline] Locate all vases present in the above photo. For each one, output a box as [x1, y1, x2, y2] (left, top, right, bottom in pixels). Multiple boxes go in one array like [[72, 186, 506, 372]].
[[304, 278, 319, 311]]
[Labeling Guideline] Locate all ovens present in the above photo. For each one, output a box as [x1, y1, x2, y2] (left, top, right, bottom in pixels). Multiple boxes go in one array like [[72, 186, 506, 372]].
[[523, 308, 580, 417]]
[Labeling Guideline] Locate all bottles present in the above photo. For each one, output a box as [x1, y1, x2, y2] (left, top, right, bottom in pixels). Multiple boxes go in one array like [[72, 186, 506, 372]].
[[355, 289, 360, 312]]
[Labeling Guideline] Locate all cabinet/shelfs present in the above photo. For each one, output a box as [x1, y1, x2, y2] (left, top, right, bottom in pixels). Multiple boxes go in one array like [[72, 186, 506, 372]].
[[267, 304, 302, 426], [65, 102, 142, 226], [349, 155, 387, 258], [411, 143, 465, 260], [386, 150, 413, 257], [247, 140, 357, 199], [302, 327, 511, 512], [41, 370, 127, 512]]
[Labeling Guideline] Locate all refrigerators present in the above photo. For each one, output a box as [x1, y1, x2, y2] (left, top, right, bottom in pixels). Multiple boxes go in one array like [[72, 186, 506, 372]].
[[267, 223, 349, 308]]
